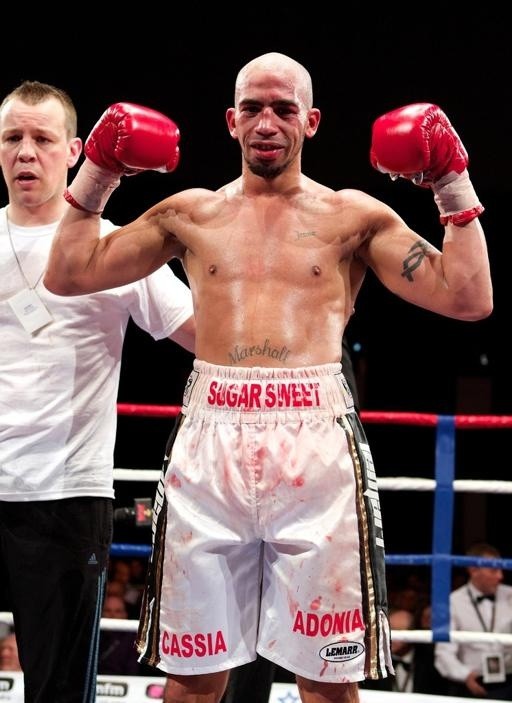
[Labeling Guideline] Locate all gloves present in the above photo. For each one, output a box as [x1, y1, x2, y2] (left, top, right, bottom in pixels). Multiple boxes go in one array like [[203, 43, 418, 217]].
[[369, 102, 484, 226], [63, 101, 180, 215]]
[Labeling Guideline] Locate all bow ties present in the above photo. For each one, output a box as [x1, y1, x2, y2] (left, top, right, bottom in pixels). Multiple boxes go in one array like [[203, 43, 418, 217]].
[[476, 593, 495, 603], [393, 659, 412, 672]]
